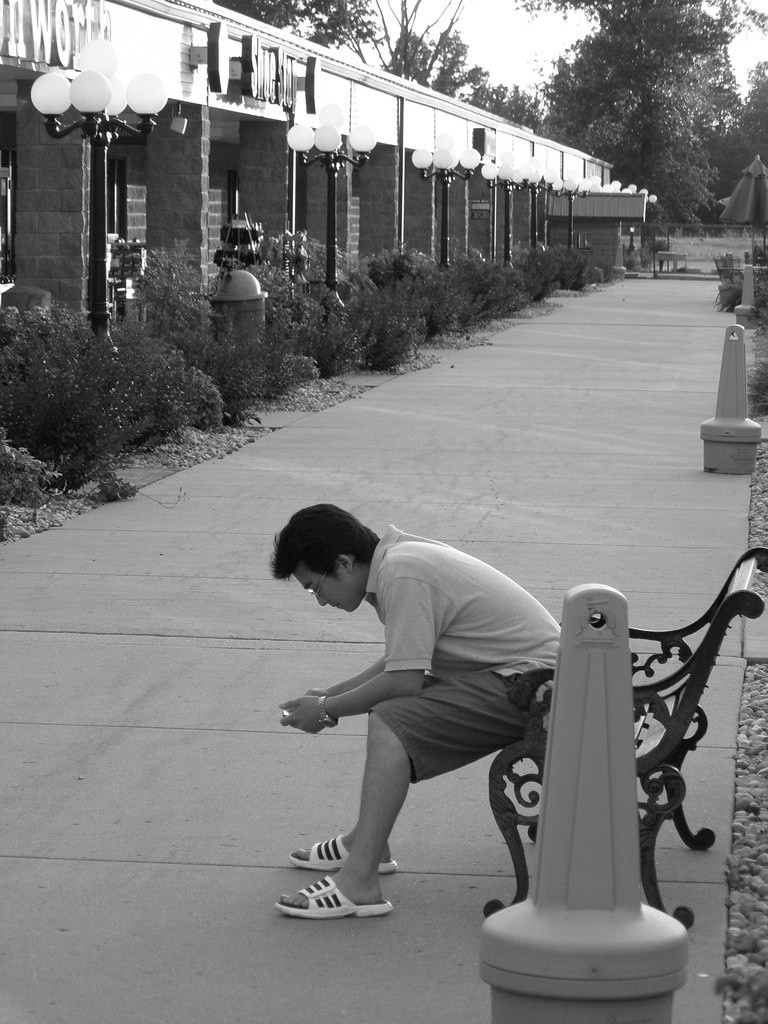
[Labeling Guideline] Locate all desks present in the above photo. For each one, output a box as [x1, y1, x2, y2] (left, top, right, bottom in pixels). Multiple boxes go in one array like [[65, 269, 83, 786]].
[[657, 251, 688, 273], [719, 267, 745, 283]]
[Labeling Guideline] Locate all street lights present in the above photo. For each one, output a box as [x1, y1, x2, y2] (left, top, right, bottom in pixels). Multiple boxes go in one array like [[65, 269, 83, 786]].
[[524, 153, 658, 258], [411, 130, 481, 278], [29, 36, 169, 361], [478, 140, 545, 274], [284, 102, 381, 321]]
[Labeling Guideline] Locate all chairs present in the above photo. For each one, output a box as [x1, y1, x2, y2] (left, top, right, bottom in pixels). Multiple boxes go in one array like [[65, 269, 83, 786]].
[[713, 253, 740, 284]]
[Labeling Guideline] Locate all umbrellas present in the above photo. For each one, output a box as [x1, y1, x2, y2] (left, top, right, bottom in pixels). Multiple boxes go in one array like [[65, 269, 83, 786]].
[[720, 151, 768, 268]]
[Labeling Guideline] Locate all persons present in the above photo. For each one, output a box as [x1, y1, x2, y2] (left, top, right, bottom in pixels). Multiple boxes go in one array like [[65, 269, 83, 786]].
[[272, 503, 560, 920]]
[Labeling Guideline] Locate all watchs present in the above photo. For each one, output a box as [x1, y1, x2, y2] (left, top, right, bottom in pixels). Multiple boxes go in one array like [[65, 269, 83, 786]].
[[317, 696, 338, 728]]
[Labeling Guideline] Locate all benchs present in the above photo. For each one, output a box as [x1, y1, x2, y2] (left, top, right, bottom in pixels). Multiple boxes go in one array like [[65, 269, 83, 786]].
[[483, 547, 768, 931]]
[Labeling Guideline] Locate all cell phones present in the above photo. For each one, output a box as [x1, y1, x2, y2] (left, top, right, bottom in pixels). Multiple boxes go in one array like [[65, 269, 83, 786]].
[[282, 710, 294, 717]]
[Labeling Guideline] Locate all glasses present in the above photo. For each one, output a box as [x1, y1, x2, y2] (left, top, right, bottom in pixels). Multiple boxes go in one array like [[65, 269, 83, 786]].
[[308, 573, 326, 597]]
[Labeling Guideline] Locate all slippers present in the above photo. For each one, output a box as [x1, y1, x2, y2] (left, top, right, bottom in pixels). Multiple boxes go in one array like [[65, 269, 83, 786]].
[[275, 876, 393, 919], [288, 834, 398, 873]]
